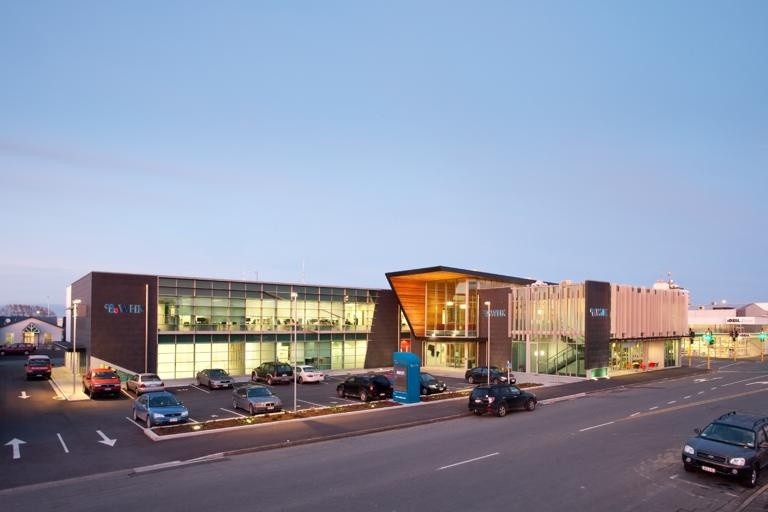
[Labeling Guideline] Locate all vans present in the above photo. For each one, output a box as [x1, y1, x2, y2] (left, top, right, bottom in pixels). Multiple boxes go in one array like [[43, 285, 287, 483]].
[[24, 354, 53, 380]]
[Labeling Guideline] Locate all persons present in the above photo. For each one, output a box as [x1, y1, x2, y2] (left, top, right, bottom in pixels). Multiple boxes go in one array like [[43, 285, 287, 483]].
[[345, 318, 352, 325], [505, 360, 512, 368], [353, 314, 358, 325]]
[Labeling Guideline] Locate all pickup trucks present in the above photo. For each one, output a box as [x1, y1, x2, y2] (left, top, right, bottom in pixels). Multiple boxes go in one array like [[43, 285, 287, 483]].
[[81, 367, 121, 399]]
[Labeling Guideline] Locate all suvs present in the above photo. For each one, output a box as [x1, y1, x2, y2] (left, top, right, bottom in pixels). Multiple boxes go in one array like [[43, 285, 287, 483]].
[[251, 361, 293, 385], [0, 342, 37, 355], [468, 383, 538, 416], [335, 374, 392, 402], [681, 411, 767, 487]]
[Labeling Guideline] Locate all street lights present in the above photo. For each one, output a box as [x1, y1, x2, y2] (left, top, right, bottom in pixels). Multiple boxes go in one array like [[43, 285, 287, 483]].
[[46, 295, 50, 316], [71, 298, 82, 395], [483, 299, 493, 385], [289, 291, 299, 413]]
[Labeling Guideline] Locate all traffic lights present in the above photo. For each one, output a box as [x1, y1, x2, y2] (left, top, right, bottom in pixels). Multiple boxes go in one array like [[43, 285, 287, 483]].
[[734, 330, 738, 337], [704, 331, 711, 340], [761, 333, 765, 341], [691, 331, 695, 344], [729, 330, 733, 337], [709, 338, 715, 345]]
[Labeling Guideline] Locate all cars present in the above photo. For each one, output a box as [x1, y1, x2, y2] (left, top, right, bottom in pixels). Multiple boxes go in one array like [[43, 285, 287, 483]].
[[419, 371, 447, 395], [464, 365, 516, 385], [130, 391, 190, 428], [292, 365, 325, 384], [231, 384, 283, 415], [196, 368, 234, 390], [126, 372, 165, 396]]
